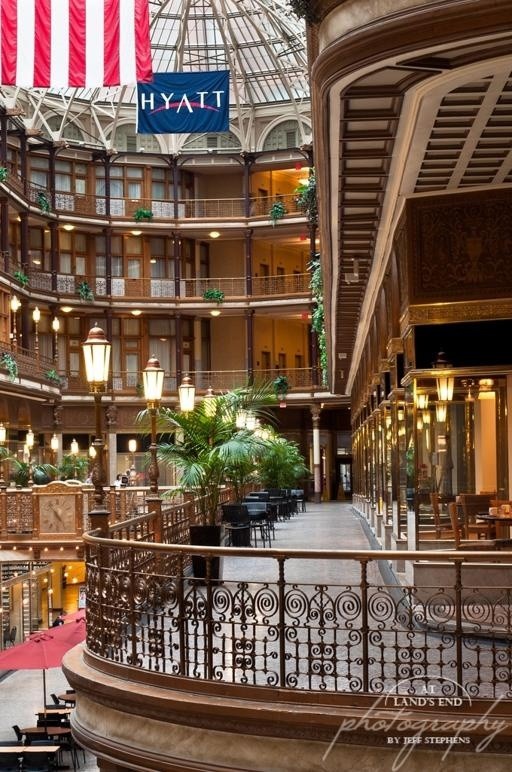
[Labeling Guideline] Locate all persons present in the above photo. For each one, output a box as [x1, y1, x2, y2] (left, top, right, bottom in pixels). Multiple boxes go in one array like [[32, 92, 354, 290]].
[[3, 626, 18, 650], [114, 465, 138, 487]]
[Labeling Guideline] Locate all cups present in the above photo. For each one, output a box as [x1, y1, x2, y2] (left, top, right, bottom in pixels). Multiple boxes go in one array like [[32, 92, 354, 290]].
[[489, 505, 511, 517]]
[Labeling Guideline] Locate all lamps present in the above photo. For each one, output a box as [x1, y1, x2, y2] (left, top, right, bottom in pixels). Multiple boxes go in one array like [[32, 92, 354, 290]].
[[0, 422, 98, 461], [128, 438, 141, 473], [79, 318, 124, 513], [178, 373, 196, 492], [7, 295, 61, 360], [139, 353, 169, 500]]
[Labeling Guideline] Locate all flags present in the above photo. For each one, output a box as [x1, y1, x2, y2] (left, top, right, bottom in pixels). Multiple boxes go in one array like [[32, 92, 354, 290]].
[[0, 1, 153, 86], [137, 68, 231, 132]]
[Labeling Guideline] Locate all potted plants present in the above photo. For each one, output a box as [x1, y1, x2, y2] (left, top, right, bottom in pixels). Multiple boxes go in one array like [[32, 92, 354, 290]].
[[135, 379, 314, 588]]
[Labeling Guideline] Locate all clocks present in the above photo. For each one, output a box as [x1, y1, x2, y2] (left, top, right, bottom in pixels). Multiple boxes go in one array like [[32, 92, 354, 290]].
[[32, 485, 83, 541]]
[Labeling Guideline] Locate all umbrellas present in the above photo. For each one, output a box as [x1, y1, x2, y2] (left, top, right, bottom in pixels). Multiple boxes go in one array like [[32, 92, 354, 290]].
[[1, 629, 77, 718], [46, 608, 89, 647]]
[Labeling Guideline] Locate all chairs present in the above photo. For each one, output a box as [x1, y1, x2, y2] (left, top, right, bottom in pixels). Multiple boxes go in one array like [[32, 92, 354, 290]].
[[447, 486, 512, 564], [0, 687, 88, 770]]
